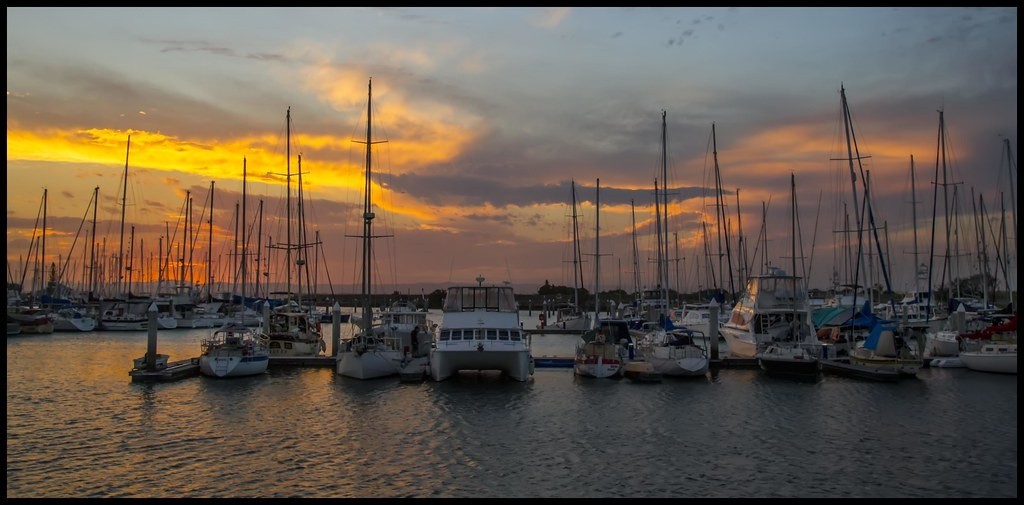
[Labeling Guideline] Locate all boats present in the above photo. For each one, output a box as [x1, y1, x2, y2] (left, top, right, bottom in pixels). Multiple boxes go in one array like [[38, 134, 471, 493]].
[[433, 272, 535, 385]]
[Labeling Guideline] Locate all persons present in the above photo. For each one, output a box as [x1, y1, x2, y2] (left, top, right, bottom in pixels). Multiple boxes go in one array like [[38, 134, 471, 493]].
[[410, 326, 420, 357]]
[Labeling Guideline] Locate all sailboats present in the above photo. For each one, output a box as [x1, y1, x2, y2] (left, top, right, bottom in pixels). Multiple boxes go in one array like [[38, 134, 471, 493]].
[[556, 81, 1018, 384], [6, 77, 437, 381]]
